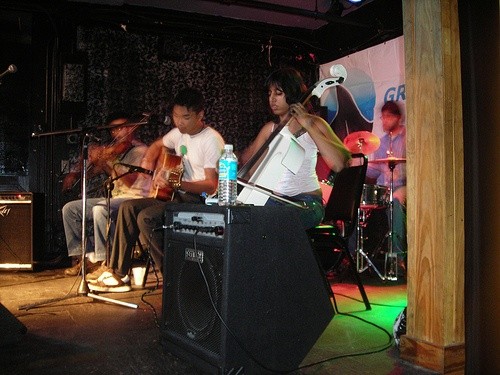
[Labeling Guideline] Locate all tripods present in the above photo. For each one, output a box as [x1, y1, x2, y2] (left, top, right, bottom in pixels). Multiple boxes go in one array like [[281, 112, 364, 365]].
[[354, 164, 407, 280], [17, 119, 155, 311]]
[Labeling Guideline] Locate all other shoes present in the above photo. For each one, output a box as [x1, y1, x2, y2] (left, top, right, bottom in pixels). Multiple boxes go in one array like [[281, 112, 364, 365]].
[[64, 258, 102, 276], [86, 266, 112, 281], [393, 261, 406, 275]]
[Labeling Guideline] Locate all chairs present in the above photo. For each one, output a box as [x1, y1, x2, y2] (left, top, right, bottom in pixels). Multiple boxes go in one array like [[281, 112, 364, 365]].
[[307, 153, 372, 315], [128, 226, 153, 289]]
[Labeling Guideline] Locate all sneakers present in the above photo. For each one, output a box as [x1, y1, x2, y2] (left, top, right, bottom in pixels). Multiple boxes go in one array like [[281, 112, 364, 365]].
[[87, 271, 132, 293]]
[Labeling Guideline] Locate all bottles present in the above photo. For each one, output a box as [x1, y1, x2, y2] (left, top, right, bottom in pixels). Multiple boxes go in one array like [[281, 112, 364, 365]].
[[218, 145, 237, 205]]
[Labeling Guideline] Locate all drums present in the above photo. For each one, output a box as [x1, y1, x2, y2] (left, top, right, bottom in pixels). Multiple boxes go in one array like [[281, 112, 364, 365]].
[[361, 183, 390, 208], [319, 178, 334, 205]]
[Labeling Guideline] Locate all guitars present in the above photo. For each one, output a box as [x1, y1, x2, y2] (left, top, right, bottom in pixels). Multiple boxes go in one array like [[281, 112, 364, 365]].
[[149, 146, 184, 201]]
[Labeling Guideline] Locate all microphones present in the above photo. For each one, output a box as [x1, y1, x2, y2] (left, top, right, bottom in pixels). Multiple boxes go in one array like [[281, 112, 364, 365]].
[[140, 112, 172, 126], [0, 64, 17, 79], [119, 162, 153, 176]]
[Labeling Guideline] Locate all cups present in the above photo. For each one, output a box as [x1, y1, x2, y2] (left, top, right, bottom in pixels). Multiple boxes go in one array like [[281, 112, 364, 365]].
[[132, 267, 146, 285]]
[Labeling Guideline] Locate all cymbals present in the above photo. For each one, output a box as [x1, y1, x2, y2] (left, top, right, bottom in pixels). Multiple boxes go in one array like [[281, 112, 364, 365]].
[[344, 131, 380, 155], [368, 157, 405, 164]]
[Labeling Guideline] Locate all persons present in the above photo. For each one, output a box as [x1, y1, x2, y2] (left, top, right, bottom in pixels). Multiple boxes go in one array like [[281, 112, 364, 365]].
[[88, 86, 226, 292], [62, 110, 156, 283], [327, 100, 407, 281], [217, 67, 347, 231]]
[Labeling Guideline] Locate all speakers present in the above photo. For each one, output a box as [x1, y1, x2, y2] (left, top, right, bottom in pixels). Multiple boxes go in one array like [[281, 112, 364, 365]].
[[0, 191, 34, 271], [0, 303, 27, 348], [159, 203, 336, 375]]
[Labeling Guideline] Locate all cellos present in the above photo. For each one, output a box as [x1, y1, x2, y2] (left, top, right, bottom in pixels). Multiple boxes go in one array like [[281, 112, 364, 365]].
[[205, 64, 347, 205]]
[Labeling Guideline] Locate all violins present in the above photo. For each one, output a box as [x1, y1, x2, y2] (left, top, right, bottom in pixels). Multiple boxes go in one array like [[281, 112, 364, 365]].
[[88, 140, 129, 163]]
